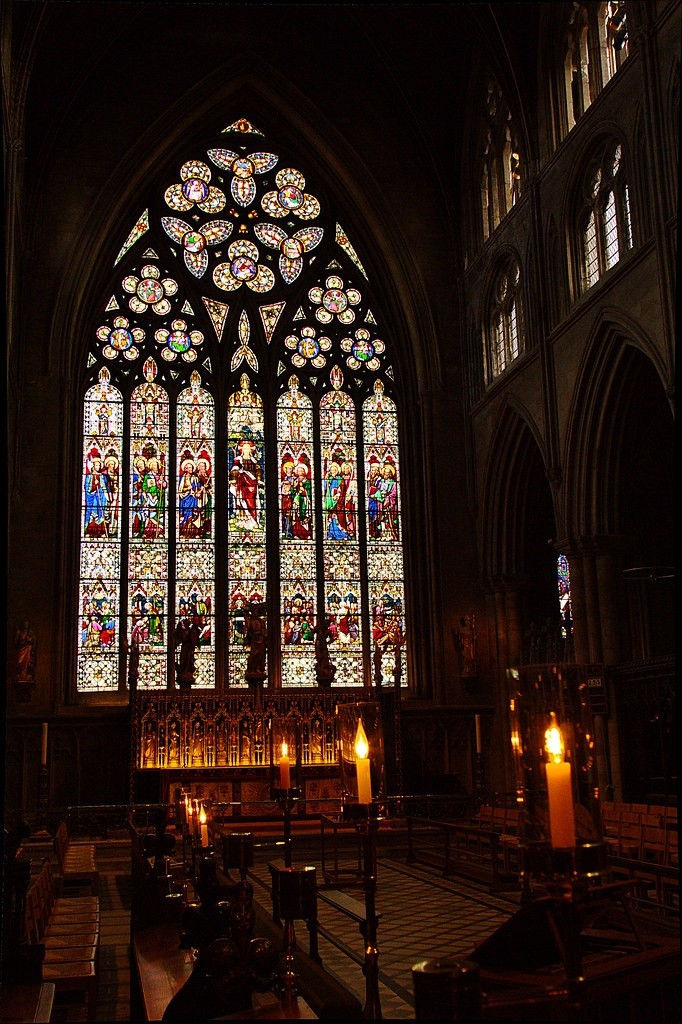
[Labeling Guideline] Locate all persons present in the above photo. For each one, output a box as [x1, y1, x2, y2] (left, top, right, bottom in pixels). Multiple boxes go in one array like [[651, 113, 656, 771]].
[[306, 619, 335, 666], [245, 608, 267, 673], [174, 619, 200, 689], [15, 619, 36, 679], [457, 616, 478, 673]]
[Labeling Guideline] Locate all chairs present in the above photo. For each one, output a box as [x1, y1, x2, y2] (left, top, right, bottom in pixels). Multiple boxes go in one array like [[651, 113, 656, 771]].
[[53, 819, 100, 898], [600, 800, 679, 920], [23, 856, 100, 1024], [455, 806, 522, 870]]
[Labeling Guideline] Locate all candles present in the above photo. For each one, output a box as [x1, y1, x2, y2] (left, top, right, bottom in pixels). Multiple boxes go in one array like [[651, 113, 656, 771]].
[[279, 740, 291, 789], [475, 715, 482, 753], [196, 803, 201, 835], [188, 799, 194, 834], [200, 803, 209, 847], [353, 717, 372, 804], [544, 714, 577, 848]]
